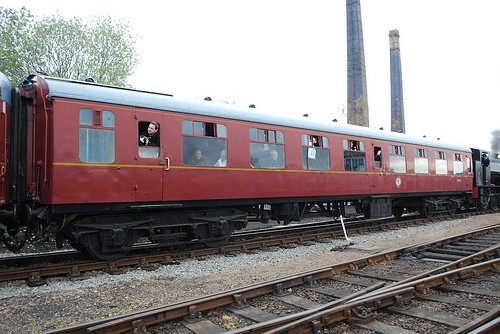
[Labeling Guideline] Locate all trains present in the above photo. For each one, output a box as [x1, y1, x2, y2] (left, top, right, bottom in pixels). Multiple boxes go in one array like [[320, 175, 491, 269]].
[[0, 71, 500, 261]]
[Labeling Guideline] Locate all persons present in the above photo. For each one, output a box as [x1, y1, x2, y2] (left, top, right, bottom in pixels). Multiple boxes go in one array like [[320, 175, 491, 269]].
[[187, 149, 211, 166], [264, 150, 285, 168], [138, 122, 159, 146], [214, 150, 227, 166]]
